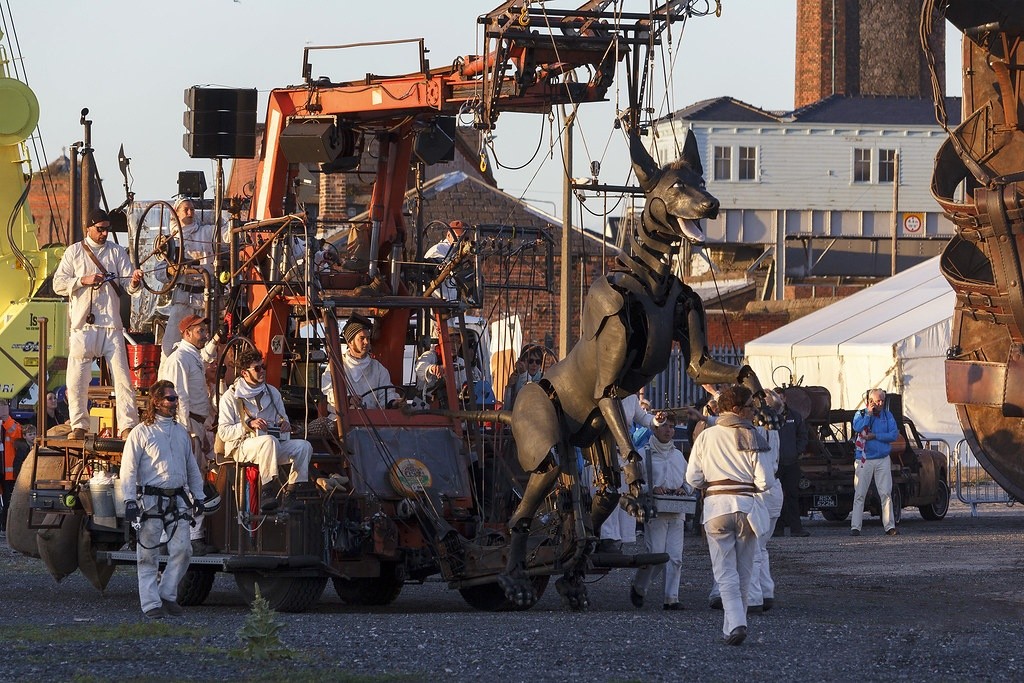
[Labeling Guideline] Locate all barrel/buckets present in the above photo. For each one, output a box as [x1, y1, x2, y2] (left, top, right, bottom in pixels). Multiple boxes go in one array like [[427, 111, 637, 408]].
[[127, 344, 162, 389], [89, 483, 115, 517]]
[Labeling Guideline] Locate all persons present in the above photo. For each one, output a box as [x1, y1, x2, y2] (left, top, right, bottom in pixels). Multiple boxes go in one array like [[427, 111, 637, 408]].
[[685, 389, 783, 615], [630, 413, 694, 611], [850, 388, 899, 535], [424, 220, 470, 301], [769, 387, 810, 538], [218, 349, 313, 509], [53, 209, 140, 440], [414, 327, 482, 403], [630, 399, 654, 449], [159, 313, 229, 556], [0, 397, 23, 538], [504, 344, 543, 410], [588, 393, 668, 543], [154, 197, 231, 365], [686, 386, 774, 644], [31, 391, 64, 430], [13, 423, 36, 477], [119, 380, 207, 618], [322, 317, 407, 422]]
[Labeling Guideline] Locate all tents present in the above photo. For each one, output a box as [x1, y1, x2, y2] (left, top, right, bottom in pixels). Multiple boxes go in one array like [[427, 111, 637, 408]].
[[746, 254, 981, 467]]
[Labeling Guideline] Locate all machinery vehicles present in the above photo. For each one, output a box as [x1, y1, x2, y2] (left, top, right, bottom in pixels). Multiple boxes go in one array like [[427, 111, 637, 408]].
[[5, 0, 723, 613]]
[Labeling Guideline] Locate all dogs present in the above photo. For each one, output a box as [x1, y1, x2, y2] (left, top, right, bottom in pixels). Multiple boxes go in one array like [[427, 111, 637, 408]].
[[392, 124, 788, 614]]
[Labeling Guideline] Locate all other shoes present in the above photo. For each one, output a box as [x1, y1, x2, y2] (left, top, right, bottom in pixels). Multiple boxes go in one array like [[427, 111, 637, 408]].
[[599, 541, 622, 554], [631, 579, 776, 645], [121, 428, 132, 440], [282, 490, 304, 510], [146, 608, 165, 619], [850, 529, 860, 536], [886, 528, 900, 535], [771, 530, 783, 537], [68, 427, 85, 440], [790, 529, 809, 536], [261, 489, 278, 511], [161, 598, 184, 615]]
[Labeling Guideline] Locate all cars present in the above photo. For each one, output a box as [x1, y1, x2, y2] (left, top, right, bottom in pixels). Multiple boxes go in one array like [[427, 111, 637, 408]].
[[793, 409, 953, 522]]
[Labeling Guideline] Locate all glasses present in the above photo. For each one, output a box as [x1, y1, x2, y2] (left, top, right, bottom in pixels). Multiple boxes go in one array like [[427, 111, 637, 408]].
[[92, 226, 113, 232], [457, 236, 469, 242], [245, 364, 266, 373], [524, 358, 542, 365], [163, 395, 179, 402], [738, 402, 754, 408]]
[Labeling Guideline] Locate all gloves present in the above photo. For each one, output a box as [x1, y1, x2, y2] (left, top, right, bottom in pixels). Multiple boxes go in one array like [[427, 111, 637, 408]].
[[125, 502, 140, 523], [193, 500, 203, 518]]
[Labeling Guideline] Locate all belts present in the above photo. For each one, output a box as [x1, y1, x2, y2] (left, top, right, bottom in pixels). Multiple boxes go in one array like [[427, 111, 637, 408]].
[[135, 486, 183, 496], [176, 284, 205, 294]]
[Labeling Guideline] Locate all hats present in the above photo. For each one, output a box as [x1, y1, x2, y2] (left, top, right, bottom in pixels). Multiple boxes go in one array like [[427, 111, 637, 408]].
[[346, 323, 368, 341], [173, 197, 191, 210], [86, 209, 112, 227], [178, 315, 212, 333], [451, 221, 470, 237], [447, 327, 460, 335]]
